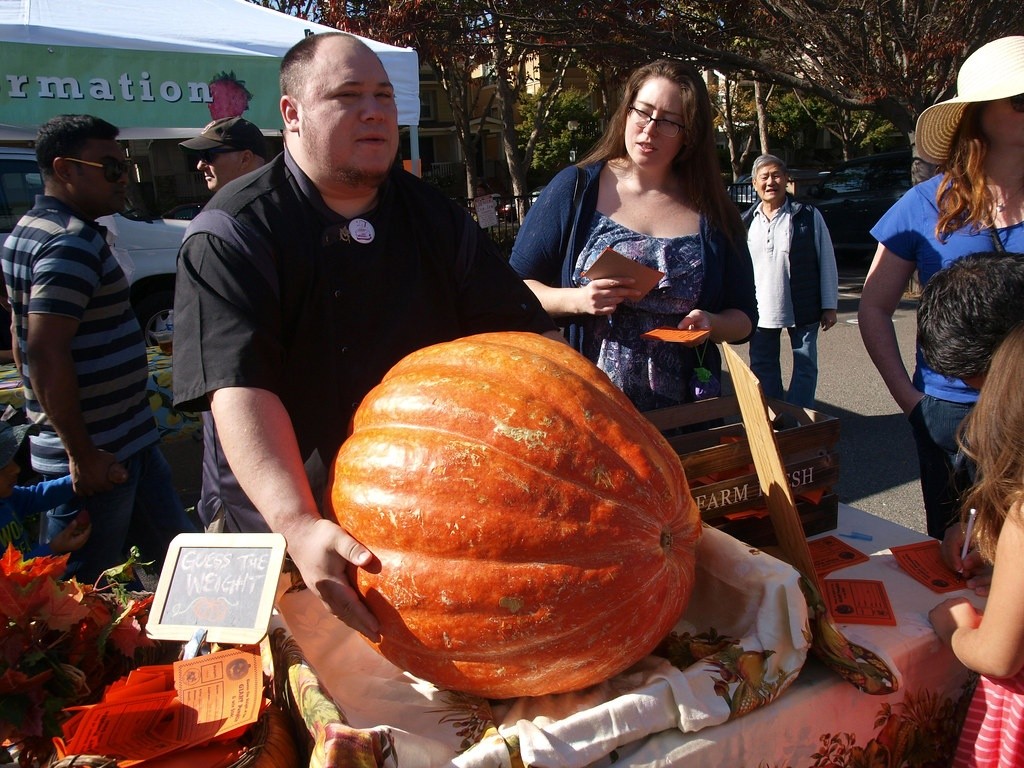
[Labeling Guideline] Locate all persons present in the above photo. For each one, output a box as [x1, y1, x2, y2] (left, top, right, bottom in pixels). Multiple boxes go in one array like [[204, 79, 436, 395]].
[[858, 35, 1024, 542], [0, 113, 269, 595], [507, 59, 759, 454], [909, 249, 1024, 768], [742, 154, 838, 431], [172, 32, 571, 641]]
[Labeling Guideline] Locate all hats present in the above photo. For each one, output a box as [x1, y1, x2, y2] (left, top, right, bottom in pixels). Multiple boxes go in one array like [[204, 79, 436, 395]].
[[914, 36, 1024, 164], [178, 115, 267, 153], [0, 420, 40, 471]]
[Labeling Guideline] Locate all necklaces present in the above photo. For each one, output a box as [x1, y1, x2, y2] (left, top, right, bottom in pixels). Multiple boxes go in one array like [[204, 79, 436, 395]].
[[996, 184, 1024, 212]]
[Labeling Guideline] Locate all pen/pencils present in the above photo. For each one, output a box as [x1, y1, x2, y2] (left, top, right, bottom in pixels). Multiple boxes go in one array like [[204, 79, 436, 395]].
[[607, 313, 615, 327], [957, 508, 977, 582]]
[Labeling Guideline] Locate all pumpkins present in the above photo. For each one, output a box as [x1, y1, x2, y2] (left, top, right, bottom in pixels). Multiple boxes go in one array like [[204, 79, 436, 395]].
[[319, 330, 700, 700]]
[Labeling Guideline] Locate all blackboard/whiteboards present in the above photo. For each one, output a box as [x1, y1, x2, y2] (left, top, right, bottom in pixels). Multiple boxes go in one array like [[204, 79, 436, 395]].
[[145, 533, 287, 645]]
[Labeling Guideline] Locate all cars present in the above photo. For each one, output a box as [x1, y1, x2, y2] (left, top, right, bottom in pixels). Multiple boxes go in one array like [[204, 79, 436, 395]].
[[0, 146, 191, 342], [727, 147, 913, 271]]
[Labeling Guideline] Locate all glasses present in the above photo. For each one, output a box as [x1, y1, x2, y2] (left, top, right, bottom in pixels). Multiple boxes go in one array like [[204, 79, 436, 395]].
[[199, 148, 245, 165], [1009, 95, 1024, 114], [626, 105, 683, 137], [60, 158, 134, 183]]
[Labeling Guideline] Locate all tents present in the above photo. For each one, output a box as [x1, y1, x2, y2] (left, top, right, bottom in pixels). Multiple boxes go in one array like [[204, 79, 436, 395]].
[[0, 0, 421, 178]]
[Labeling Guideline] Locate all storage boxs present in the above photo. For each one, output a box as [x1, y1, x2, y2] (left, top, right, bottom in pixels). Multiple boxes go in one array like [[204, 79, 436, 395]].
[[640, 394, 840, 547]]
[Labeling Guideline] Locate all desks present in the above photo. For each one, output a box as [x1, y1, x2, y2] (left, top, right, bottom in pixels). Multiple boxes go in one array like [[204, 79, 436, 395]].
[[607, 501, 992, 768], [0, 345, 207, 445]]
